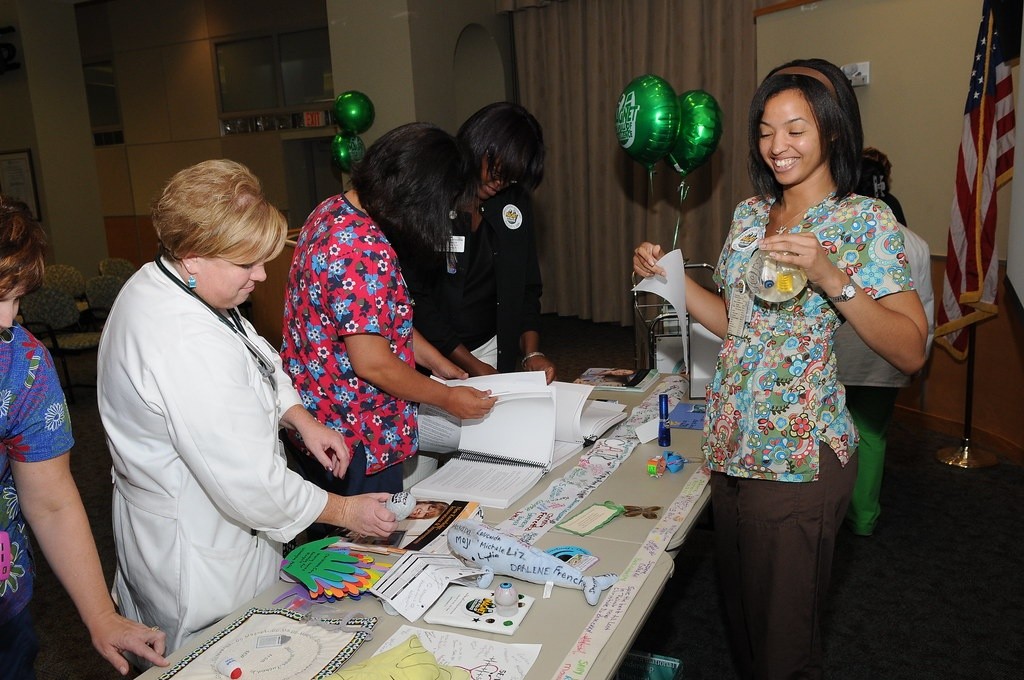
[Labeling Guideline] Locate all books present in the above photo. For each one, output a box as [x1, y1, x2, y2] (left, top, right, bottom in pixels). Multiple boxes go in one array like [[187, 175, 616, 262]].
[[321, 368, 663, 557]]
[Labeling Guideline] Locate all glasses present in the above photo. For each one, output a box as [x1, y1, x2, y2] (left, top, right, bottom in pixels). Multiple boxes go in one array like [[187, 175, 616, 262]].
[[487, 149, 518, 184]]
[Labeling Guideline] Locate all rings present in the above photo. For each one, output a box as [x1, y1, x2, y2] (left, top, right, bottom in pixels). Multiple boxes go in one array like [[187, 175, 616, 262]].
[[151, 626, 160, 632]]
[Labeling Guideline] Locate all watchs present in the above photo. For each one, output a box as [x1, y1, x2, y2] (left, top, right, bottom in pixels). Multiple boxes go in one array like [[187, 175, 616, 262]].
[[828, 275, 857, 302]]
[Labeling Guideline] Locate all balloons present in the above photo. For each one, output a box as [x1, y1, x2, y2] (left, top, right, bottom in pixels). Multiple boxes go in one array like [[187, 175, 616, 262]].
[[331, 90, 376, 174], [616, 73, 724, 178]]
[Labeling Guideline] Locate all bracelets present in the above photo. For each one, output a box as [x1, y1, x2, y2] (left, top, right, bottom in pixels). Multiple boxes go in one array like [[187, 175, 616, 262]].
[[521, 352, 546, 370]]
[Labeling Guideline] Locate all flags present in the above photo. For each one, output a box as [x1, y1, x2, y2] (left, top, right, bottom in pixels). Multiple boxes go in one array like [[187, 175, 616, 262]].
[[934, 1, 1015, 362]]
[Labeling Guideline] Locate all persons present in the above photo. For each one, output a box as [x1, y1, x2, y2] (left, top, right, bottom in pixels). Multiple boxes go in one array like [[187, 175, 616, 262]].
[[402, 102, 556, 386], [280, 123, 499, 542], [0, 196, 171, 680], [832, 148, 934, 536], [406, 501, 449, 520], [96, 159, 399, 670], [634, 58, 929, 680]]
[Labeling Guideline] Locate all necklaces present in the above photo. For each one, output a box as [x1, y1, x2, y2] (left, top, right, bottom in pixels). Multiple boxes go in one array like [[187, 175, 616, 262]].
[[776, 198, 821, 235]]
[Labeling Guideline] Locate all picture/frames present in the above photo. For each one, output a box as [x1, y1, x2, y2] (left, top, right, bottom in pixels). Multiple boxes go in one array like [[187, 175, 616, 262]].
[[0, 148, 41, 226]]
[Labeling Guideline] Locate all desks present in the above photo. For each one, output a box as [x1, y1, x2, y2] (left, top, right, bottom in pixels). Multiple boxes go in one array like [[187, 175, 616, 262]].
[[132, 366, 711, 680]]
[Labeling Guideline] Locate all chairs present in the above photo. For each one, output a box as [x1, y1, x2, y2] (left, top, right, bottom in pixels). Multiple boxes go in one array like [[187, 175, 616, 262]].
[[14, 257, 147, 385]]
